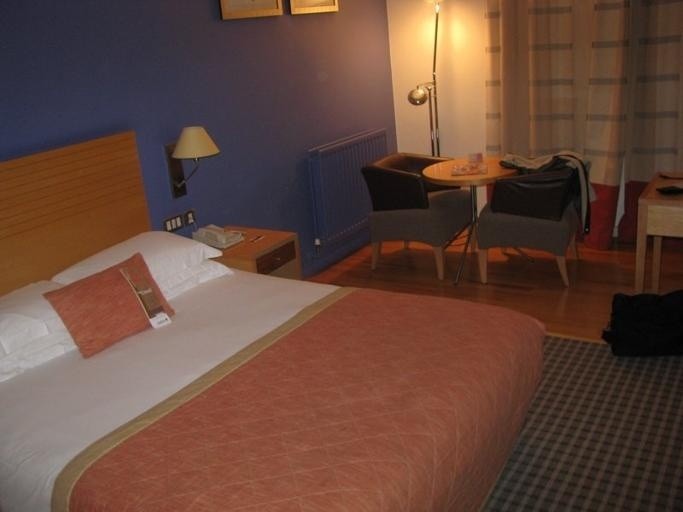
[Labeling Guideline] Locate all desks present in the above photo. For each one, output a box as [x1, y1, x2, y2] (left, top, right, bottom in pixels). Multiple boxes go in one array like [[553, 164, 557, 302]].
[[634, 170, 681, 294], [421, 156, 519, 287]]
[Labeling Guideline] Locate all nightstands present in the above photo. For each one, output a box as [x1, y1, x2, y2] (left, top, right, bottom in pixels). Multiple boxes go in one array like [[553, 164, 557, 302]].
[[207, 225, 301, 280]]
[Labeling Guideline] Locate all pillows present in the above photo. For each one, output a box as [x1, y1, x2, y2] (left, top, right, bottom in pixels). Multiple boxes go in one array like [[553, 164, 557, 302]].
[[50, 230, 222, 286], [42, 252, 175, 359], [157, 258, 234, 301], [1, 280, 78, 382]]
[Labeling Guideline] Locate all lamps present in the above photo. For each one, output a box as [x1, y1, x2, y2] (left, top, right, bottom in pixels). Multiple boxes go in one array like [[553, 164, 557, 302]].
[[407, 1, 442, 157], [165, 127, 220, 200]]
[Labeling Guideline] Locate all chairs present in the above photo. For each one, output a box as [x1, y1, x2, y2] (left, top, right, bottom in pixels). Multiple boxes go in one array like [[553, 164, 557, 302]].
[[359, 152, 466, 281], [475, 153, 592, 288]]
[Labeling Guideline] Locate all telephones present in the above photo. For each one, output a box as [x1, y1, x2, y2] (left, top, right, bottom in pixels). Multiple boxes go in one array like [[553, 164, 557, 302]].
[[191, 223, 245, 249]]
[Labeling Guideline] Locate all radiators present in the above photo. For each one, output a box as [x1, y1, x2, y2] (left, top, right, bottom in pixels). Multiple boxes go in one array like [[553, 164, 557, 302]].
[[306, 125, 400, 258]]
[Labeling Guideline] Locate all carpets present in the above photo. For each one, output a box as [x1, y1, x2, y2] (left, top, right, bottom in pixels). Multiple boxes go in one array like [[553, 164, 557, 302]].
[[483, 331, 681, 510]]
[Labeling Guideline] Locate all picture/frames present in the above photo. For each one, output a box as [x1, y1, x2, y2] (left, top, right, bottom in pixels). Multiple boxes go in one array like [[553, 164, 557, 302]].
[[290, 0, 339, 15], [220, 0, 283, 21]]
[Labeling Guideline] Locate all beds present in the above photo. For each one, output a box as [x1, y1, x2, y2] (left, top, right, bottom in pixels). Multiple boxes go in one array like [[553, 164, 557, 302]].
[[1, 130, 546, 511]]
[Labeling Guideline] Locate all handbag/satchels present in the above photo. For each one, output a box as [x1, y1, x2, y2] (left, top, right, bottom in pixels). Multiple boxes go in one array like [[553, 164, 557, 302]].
[[601, 291, 682, 357]]
